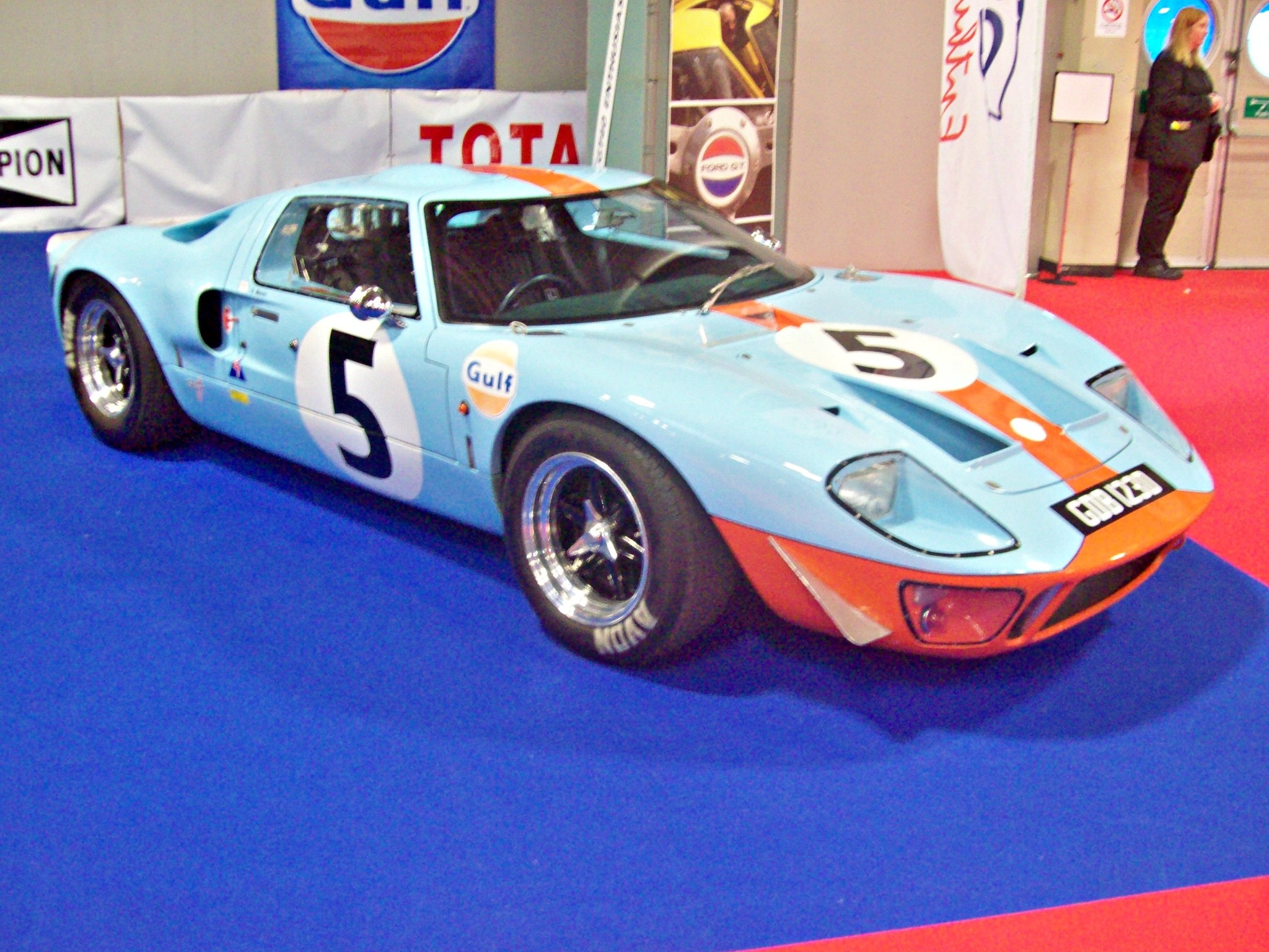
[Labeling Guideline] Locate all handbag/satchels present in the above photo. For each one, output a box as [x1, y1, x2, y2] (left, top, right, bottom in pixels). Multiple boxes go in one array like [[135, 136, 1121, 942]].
[[1135, 111, 1211, 171]]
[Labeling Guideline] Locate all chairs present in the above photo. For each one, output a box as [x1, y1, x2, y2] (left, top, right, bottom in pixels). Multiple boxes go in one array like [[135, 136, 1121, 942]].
[[347, 225, 452, 315], [459, 213, 554, 310]]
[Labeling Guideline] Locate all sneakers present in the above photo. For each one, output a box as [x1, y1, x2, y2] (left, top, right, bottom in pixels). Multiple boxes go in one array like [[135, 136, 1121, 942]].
[[1130, 260, 1184, 280]]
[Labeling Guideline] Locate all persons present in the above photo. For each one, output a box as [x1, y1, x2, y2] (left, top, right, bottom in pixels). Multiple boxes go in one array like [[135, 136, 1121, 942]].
[[1132, 6, 1226, 281]]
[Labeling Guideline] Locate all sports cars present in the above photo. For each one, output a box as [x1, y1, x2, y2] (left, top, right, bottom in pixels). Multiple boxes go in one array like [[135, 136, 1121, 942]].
[[47, 162, 1221, 669], [668, 0, 779, 248]]
